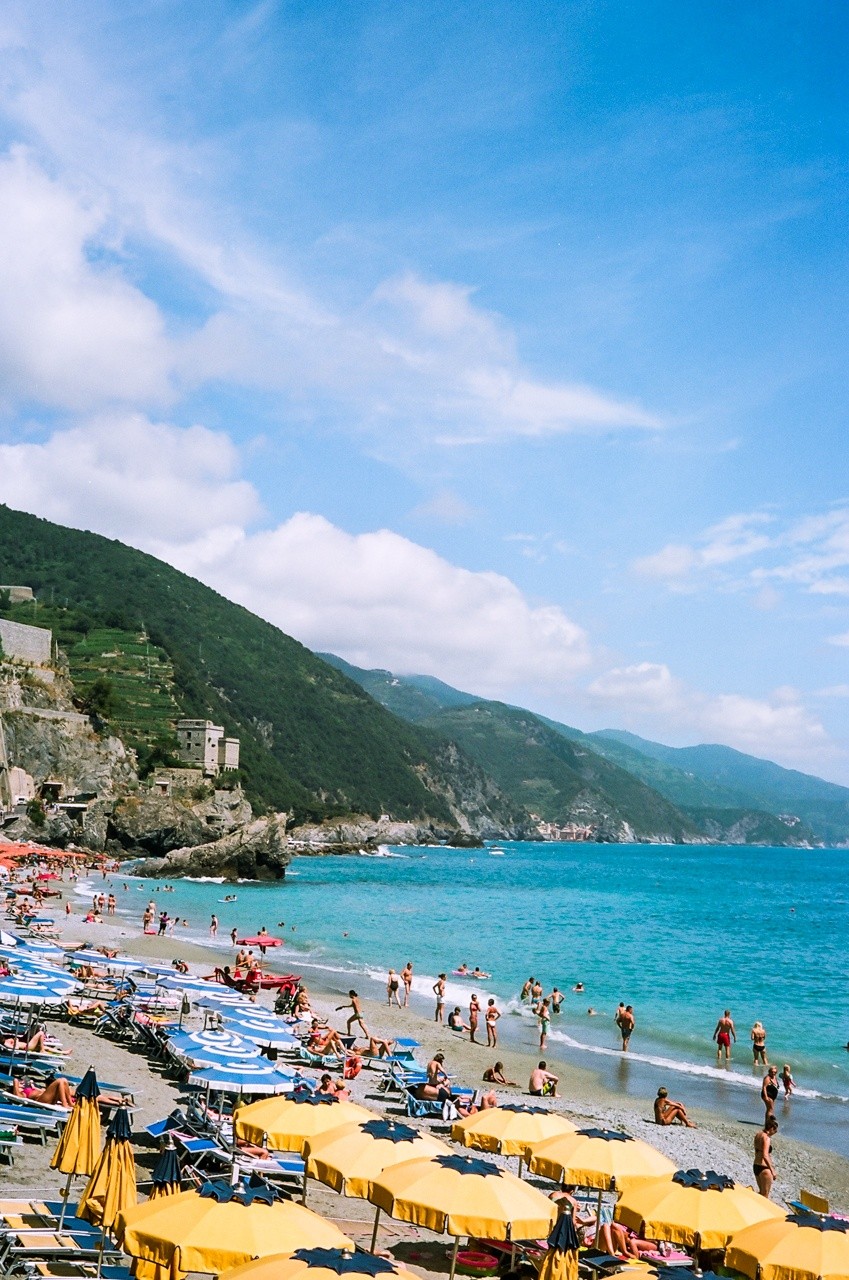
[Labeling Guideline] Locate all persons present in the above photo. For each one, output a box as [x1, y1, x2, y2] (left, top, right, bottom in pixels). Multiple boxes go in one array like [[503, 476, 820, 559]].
[[335, 990, 369, 1039], [653, 1087, 698, 1129], [529, 1060, 561, 1097], [0, 839, 795, 1259], [483, 1062, 516, 1087]]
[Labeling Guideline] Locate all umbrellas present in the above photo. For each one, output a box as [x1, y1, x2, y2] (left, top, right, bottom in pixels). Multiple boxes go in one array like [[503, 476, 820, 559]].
[[0, 927, 849, 1280]]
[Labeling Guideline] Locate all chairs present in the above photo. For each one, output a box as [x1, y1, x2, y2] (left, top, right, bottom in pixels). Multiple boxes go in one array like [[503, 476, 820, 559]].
[[0, 968, 715, 1280], [4, 883, 92, 951]]
